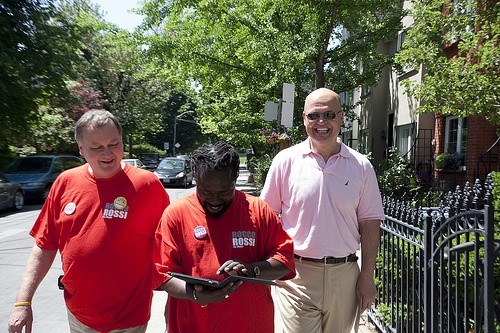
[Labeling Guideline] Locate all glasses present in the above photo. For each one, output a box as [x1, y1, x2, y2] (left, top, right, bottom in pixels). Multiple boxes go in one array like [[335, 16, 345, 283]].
[[305, 109, 342, 120]]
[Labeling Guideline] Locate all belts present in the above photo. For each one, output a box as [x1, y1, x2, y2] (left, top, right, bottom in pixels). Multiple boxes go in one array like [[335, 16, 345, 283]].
[[294, 253, 359, 265]]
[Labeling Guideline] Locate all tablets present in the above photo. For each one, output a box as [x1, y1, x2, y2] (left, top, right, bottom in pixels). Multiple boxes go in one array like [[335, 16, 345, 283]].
[[171, 271, 218, 284]]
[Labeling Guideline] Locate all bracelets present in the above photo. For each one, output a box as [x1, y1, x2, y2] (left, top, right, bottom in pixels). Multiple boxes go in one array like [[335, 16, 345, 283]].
[[192, 290, 208, 307], [15, 301, 31, 306]]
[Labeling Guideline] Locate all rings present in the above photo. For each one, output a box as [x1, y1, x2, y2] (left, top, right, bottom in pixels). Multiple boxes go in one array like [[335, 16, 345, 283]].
[[224, 294, 229, 298]]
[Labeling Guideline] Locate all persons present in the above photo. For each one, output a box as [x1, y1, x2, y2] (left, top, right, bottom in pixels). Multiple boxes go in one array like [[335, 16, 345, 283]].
[[8, 109, 172, 333], [258, 88, 386, 333], [149, 141, 296, 333]]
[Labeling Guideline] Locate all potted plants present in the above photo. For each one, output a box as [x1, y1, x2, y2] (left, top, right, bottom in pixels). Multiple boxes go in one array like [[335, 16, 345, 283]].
[[434, 153, 459, 180]]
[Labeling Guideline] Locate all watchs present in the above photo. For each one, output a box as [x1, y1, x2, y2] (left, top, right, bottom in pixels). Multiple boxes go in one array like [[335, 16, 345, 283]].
[[251, 264, 260, 277]]
